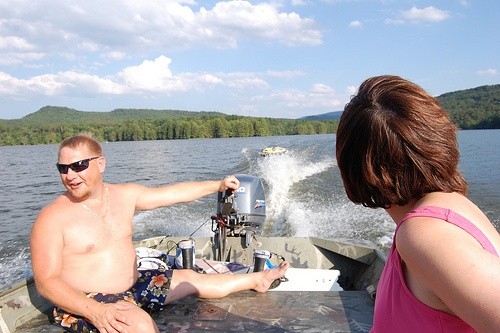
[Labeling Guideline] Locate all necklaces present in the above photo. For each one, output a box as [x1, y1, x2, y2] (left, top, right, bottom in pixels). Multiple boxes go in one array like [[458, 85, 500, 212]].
[[77, 183, 111, 225]]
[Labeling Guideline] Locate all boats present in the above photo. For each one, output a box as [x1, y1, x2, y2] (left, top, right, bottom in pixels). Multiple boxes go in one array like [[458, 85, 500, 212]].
[[258, 147, 288, 157]]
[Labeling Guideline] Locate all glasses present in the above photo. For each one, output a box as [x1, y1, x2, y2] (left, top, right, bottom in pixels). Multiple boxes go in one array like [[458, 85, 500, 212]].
[[56, 156, 100, 174]]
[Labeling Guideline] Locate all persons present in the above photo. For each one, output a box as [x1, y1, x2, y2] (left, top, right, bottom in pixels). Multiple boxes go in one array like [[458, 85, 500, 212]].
[[31, 134, 241, 333], [335, 74, 500, 333]]
